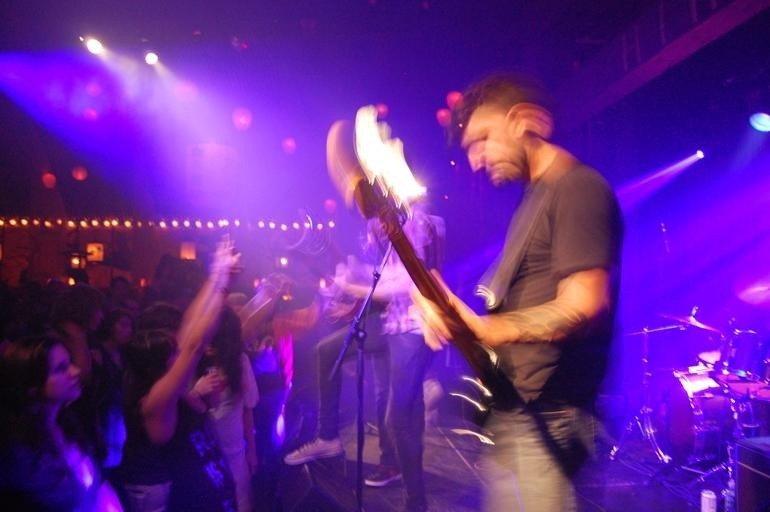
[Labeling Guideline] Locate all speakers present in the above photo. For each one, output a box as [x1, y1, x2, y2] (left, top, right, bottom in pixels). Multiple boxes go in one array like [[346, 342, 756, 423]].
[[734, 437, 770, 512], [280, 457, 365, 512]]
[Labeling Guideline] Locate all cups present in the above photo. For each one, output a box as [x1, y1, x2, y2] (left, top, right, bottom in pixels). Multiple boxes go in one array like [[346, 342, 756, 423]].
[[701, 489, 717, 512]]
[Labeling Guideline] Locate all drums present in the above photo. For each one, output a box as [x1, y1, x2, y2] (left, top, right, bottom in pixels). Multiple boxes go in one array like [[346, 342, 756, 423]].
[[660, 367, 730, 465], [715, 372, 769, 435]]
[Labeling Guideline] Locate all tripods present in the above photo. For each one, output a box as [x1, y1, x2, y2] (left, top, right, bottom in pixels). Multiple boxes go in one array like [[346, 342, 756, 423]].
[[608, 324, 672, 464]]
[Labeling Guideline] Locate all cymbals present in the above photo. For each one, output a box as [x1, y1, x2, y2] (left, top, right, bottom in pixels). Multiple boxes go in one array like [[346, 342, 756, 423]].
[[661, 312, 719, 333], [700, 348, 769, 367]]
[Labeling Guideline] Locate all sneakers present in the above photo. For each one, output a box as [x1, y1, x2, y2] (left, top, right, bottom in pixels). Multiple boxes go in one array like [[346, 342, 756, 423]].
[[284, 434, 347, 465], [362, 461, 405, 489]]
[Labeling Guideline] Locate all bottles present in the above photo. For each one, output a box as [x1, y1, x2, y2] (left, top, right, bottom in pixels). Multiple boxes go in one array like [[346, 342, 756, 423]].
[[725, 478, 735, 512]]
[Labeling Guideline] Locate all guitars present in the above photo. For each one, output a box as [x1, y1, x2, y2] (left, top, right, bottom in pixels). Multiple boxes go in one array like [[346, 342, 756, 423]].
[[327, 102, 511, 424]]
